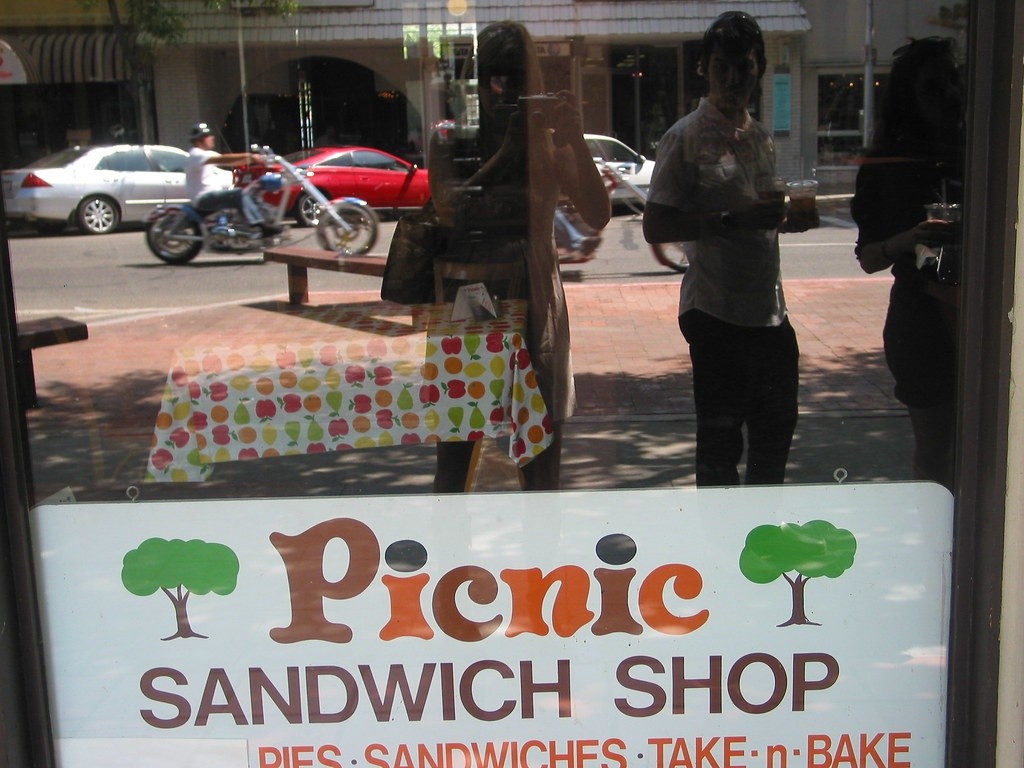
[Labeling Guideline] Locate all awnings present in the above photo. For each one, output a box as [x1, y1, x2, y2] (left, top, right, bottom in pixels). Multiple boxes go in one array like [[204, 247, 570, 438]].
[[0, 30, 147, 83]]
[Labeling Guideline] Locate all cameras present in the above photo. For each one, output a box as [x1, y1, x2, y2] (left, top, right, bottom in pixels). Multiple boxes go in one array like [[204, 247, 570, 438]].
[[517, 93, 560, 126]]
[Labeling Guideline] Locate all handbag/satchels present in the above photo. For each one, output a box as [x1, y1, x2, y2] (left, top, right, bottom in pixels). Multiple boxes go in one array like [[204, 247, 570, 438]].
[[380, 197, 478, 305]]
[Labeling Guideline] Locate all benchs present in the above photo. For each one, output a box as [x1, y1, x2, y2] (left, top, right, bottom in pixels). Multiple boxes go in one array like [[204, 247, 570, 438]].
[[266, 246, 389, 305], [16, 316, 89, 408]]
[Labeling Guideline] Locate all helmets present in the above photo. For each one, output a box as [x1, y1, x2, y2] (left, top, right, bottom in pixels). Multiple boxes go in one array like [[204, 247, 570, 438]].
[[189, 122, 218, 140]]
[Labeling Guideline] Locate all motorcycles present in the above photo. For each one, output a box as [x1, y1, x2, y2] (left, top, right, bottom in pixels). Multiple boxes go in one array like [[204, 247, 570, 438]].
[[555, 160, 699, 272], [145, 144, 379, 267]]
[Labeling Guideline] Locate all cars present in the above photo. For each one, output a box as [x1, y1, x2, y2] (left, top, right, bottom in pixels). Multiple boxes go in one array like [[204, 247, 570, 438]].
[[578, 134, 659, 202], [229, 144, 432, 228], [0, 143, 234, 235]]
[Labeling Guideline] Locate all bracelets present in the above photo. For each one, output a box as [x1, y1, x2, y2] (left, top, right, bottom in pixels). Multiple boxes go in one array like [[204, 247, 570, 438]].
[[782, 218, 792, 232], [250, 153, 253, 161]]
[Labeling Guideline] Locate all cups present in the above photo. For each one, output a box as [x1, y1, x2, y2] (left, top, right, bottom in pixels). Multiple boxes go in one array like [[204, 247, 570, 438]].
[[787, 179, 818, 214], [924, 203, 959, 253]]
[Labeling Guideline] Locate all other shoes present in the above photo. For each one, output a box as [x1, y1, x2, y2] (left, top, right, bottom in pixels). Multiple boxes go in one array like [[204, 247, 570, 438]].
[[249, 223, 282, 238]]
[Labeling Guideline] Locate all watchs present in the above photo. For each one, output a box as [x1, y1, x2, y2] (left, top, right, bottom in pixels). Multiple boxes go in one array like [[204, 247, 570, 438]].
[[721, 211, 732, 227]]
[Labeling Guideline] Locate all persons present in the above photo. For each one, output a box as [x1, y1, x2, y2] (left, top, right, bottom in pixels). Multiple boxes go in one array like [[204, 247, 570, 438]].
[[187, 123, 283, 240], [643, 12, 820, 487], [850, 36, 966, 491], [430, 21, 611, 492]]
